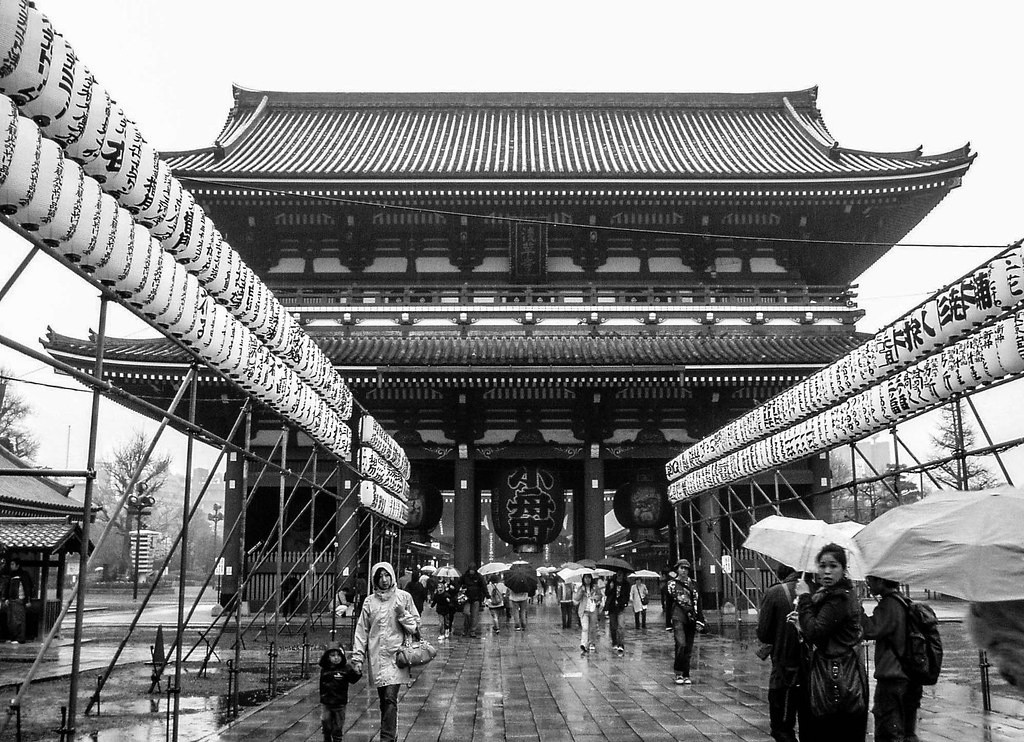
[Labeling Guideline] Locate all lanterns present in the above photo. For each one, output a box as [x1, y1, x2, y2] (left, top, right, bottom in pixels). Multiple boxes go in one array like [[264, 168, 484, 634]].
[[1, 1, 413, 524], [665, 244, 1023, 503], [491, 463, 566, 552]]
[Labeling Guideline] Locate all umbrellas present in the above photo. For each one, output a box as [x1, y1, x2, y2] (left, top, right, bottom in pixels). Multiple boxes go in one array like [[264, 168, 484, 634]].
[[833, 519, 866, 541], [503, 564, 539, 595], [627, 570, 661, 578], [556, 568, 572, 581], [535, 566, 560, 576], [432, 562, 462, 579], [477, 562, 510, 576], [595, 557, 635, 571], [743, 515, 863, 619], [576, 558, 598, 567], [594, 569, 615, 577], [565, 568, 600, 583], [421, 566, 436, 572], [853, 487, 1023, 602]]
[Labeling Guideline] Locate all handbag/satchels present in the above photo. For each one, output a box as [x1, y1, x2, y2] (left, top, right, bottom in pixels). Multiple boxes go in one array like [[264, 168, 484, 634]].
[[810, 652, 869, 719], [396, 628, 437, 669], [641, 595, 650, 605], [457, 592, 468, 605]]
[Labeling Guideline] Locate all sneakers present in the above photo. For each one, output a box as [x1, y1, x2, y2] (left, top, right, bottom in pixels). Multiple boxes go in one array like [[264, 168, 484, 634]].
[[684, 677, 691, 684], [675, 675, 683, 684]]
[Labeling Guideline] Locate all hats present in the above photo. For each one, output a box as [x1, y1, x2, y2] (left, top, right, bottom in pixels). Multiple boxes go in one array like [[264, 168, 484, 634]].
[[674, 559, 690, 567]]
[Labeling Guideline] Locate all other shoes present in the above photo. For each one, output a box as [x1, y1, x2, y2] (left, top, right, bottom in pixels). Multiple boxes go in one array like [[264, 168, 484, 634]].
[[618, 646, 623, 651], [613, 645, 618, 650], [589, 644, 596, 649], [580, 644, 588, 652], [445, 629, 451, 638], [438, 634, 445, 640]]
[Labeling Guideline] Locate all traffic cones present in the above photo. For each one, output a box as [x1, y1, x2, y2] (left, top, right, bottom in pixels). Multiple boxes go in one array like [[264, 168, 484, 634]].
[[142, 623, 173, 666]]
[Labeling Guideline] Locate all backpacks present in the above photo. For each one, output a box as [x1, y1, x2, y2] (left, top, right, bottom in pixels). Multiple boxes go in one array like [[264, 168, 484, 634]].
[[491, 585, 503, 604], [887, 593, 943, 686]]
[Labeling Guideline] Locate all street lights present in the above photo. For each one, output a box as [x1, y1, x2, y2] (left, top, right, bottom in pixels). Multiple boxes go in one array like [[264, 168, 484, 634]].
[[207, 502, 224, 590], [124, 482, 157, 601]]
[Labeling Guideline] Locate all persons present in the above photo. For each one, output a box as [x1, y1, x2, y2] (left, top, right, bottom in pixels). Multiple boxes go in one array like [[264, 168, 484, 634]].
[[536, 573, 560, 605], [859, 574, 923, 741], [319, 640, 361, 742], [352, 562, 422, 742], [785, 545, 869, 742], [400, 570, 533, 640], [660, 571, 676, 632], [555, 574, 650, 653], [665, 558, 705, 685], [761, 561, 818, 742]]
